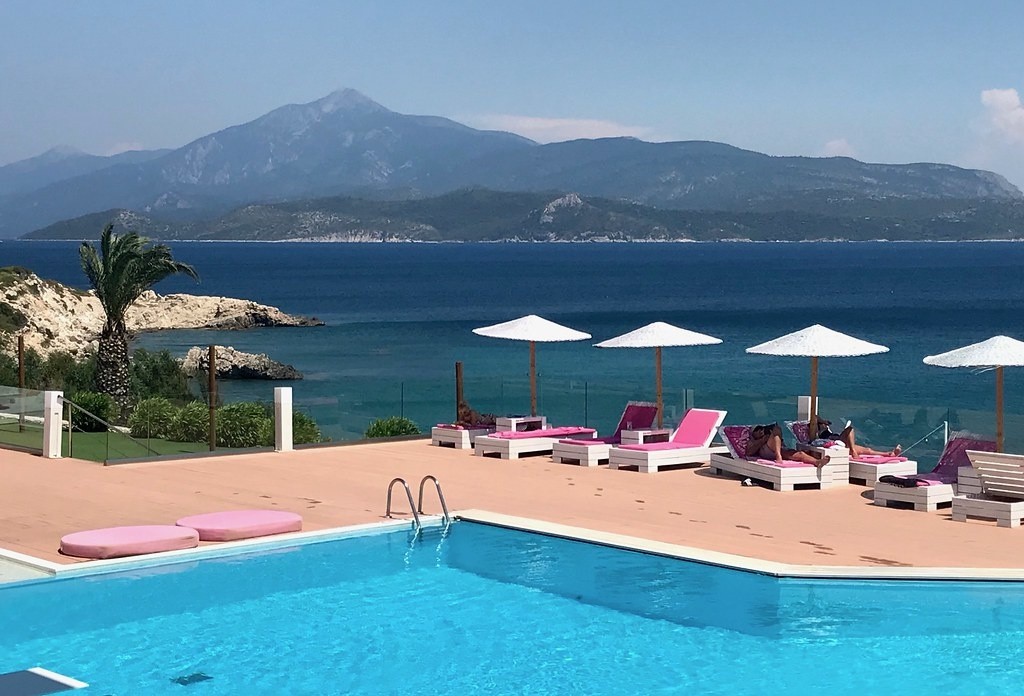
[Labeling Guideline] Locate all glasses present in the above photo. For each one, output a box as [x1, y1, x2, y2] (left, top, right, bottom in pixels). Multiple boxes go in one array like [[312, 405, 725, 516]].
[[458, 411, 467, 415], [755, 427, 764, 432]]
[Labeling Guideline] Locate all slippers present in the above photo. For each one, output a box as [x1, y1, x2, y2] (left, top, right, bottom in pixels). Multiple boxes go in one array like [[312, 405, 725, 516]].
[[742, 478, 759, 486]]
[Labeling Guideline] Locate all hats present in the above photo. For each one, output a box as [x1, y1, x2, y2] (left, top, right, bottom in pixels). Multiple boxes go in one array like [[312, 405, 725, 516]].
[[750, 424, 766, 433]]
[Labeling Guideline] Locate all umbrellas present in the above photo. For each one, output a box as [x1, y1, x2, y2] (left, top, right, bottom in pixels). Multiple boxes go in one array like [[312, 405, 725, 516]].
[[923, 335, 1024, 452], [593, 321, 723, 430], [471, 315, 593, 416], [745, 324, 891, 441]]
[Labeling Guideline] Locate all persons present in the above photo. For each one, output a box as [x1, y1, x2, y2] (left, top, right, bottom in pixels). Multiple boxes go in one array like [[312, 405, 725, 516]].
[[808, 416, 901, 459], [457, 401, 526, 426], [746, 424, 831, 467]]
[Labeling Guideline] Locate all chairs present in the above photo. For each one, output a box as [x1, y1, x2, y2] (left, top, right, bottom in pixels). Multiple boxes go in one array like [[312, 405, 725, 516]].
[[874, 431, 1005, 511], [710, 423, 833, 491], [952, 449, 1024, 528], [474, 426, 598, 460], [431, 423, 496, 449], [783, 420, 917, 488], [609, 408, 730, 473], [552, 400, 659, 467]]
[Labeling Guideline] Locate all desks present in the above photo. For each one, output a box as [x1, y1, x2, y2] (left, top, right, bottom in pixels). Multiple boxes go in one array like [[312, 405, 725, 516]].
[[496, 416, 547, 431], [621, 429, 673, 444], [796, 442, 849, 490], [957, 465, 984, 496]]
